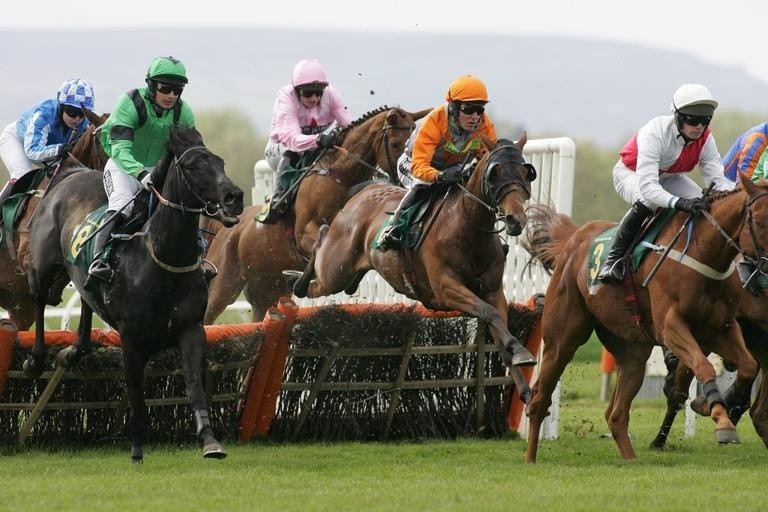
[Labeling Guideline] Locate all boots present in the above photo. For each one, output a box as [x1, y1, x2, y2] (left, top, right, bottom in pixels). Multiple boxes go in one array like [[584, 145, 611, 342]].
[[378, 198, 416, 253], [598, 200, 649, 283], [274, 157, 291, 215], [83, 211, 124, 291]]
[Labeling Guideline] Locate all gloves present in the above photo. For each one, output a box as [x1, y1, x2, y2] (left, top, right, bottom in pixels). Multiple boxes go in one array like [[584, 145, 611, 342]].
[[675, 197, 711, 216], [438, 165, 462, 183], [137, 171, 153, 192], [317, 134, 342, 149], [58, 143, 73, 158]]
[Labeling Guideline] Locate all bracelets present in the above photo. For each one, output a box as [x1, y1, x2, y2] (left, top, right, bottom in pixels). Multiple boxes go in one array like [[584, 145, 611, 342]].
[[437, 170, 442, 182]]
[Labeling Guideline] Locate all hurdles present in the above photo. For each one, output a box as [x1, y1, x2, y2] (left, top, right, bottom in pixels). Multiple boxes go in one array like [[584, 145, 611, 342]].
[[1, 294, 546, 443]]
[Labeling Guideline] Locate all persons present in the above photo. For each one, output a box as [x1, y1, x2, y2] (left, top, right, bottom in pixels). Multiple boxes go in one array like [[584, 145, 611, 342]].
[[719, 119, 768, 181], [253, 57, 352, 224], [597, 83, 737, 285], [0, 77, 94, 206], [83, 55, 196, 291], [376, 74, 496, 252]]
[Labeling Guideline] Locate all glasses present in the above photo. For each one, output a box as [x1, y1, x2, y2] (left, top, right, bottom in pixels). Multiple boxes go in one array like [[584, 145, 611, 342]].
[[300, 89, 323, 97], [461, 103, 484, 115], [63, 104, 85, 119], [681, 115, 711, 126], [155, 82, 183, 95]]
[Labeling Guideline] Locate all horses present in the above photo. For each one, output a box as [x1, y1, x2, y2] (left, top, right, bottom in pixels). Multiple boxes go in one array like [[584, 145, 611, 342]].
[[0, 100, 111, 331], [22, 119, 244, 465], [280, 130, 551, 417], [511, 168, 768, 465], [201, 104, 434, 325]]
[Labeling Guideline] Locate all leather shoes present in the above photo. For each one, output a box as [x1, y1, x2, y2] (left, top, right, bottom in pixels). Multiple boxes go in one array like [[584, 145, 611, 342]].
[[737, 262, 761, 297]]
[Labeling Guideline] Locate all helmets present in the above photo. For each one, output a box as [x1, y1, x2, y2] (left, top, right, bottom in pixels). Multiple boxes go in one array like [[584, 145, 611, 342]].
[[447, 76, 489, 104], [57, 79, 94, 110], [672, 83, 718, 113], [293, 59, 328, 88], [145, 57, 188, 84]]
